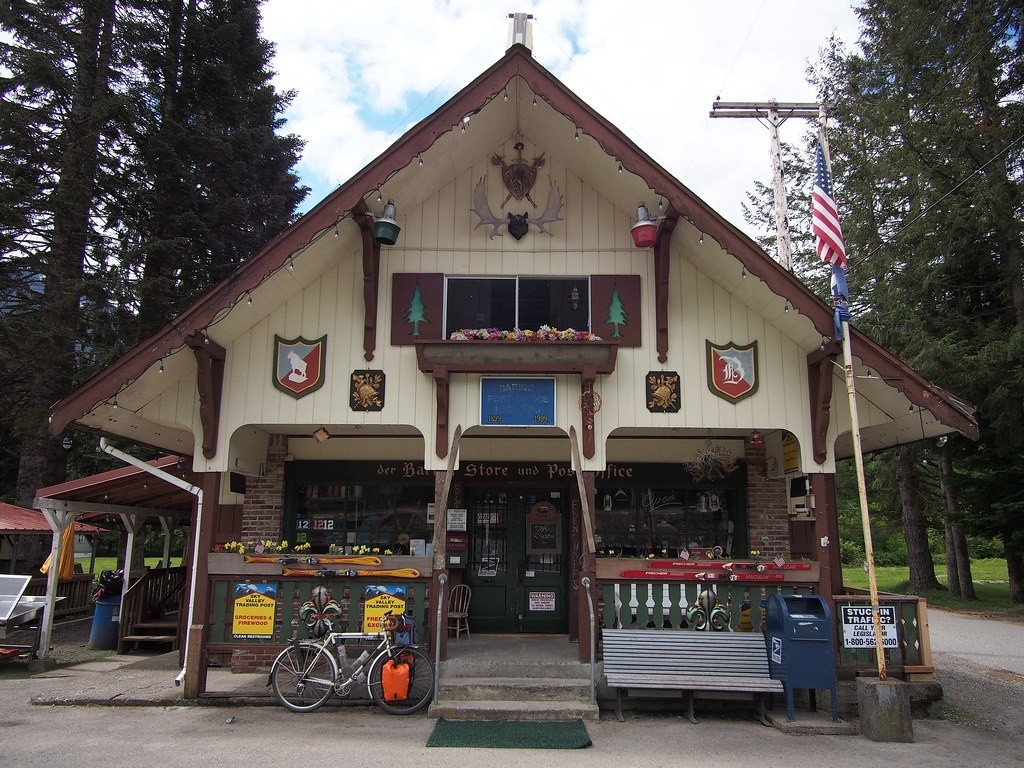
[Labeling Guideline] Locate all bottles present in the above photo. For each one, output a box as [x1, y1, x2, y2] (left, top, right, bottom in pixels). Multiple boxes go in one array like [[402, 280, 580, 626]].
[[603, 493, 612, 511], [352, 650, 370, 671], [709, 493, 719, 511], [337, 645, 349, 669]]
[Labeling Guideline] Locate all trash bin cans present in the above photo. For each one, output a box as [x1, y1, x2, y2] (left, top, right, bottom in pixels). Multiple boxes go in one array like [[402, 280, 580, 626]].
[[86, 600, 119, 651], [761, 593, 839, 722]]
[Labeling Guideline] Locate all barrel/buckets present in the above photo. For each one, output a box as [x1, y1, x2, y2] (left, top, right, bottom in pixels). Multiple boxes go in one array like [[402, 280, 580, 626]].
[[88, 596, 120, 651]]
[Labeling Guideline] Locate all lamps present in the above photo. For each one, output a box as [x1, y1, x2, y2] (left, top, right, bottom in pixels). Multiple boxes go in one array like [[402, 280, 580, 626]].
[[363, 199, 402, 245], [630, 202, 669, 247], [681, 429, 739, 482], [749, 430, 762, 447], [312, 427, 330, 443]]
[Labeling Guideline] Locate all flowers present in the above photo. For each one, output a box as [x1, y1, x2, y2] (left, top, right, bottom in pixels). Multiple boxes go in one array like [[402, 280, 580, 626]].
[[329, 544, 393, 556], [410, 546, 415, 555], [750, 550, 760, 559], [451, 324, 602, 340], [213, 539, 311, 554], [707, 548, 721, 560], [609, 550, 615, 558], [661, 549, 669, 557], [648, 554, 654, 559]]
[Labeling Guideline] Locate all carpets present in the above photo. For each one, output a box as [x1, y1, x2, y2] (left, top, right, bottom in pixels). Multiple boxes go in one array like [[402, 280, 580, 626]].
[[425, 716, 592, 749]]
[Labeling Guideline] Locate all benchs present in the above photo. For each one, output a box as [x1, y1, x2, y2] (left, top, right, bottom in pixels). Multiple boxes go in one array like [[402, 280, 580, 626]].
[[602, 628, 784, 727]]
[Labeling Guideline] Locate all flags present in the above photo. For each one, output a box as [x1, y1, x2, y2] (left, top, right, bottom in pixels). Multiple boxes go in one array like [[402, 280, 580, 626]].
[[810, 125, 849, 271]]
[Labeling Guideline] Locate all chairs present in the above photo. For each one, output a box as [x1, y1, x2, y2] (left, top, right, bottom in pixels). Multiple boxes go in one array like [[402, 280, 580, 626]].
[[448, 585, 472, 642]]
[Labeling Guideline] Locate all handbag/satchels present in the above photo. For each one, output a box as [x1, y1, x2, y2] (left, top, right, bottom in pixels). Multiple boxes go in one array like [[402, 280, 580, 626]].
[[380, 647, 416, 702], [392, 614, 417, 645], [92, 569, 123, 602]]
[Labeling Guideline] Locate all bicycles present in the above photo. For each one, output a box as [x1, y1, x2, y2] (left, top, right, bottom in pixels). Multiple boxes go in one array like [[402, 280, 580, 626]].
[[267, 605, 436, 715]]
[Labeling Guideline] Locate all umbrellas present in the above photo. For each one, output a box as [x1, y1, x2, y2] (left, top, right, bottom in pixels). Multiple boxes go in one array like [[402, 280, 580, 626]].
[[39, 516, 75, 581]]
